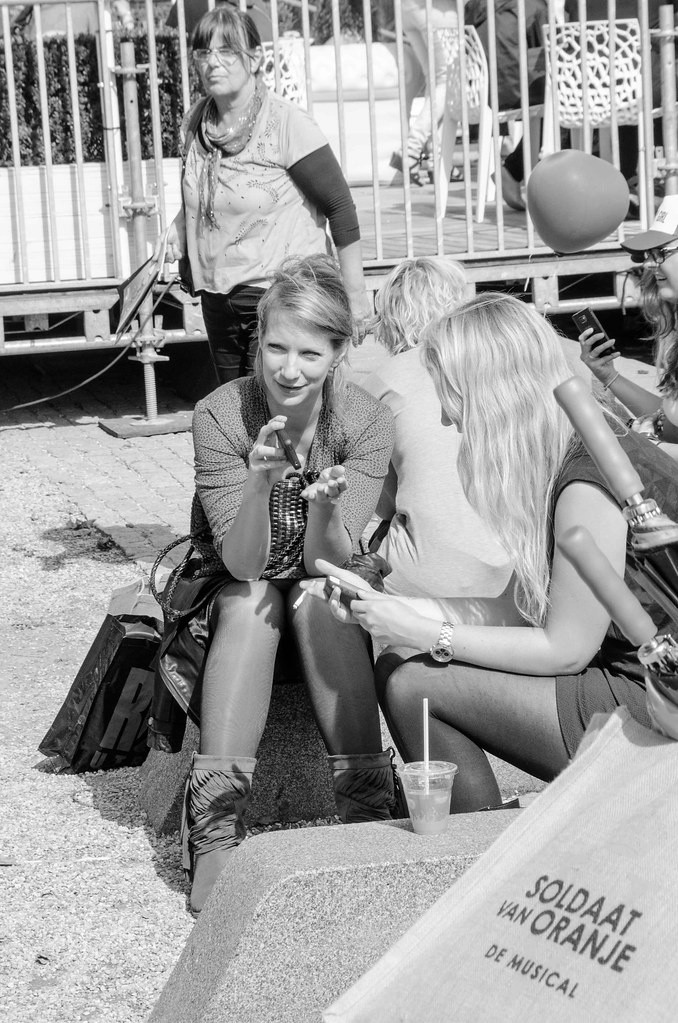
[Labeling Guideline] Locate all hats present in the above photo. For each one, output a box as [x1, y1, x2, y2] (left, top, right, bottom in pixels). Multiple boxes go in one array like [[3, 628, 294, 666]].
[[621, 195, 678, 255]]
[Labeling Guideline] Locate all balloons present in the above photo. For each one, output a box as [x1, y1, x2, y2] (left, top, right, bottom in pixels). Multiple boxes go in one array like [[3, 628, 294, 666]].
[[526, 148, 630, 255]]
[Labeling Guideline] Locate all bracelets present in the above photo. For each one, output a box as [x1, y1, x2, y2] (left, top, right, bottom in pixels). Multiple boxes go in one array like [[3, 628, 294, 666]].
[[603, 371, 619, 391]]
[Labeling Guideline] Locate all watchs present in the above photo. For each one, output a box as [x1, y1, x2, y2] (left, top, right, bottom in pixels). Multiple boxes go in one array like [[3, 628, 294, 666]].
[[429, 621, 455, 663]]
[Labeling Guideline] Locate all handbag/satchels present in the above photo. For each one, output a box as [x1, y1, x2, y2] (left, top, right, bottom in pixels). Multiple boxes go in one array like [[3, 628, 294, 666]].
[[38, 577, 179, 774]]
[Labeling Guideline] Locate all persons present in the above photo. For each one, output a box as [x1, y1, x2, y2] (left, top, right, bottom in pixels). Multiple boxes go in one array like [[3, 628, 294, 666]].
[[185, 254, 400, 920], [390, 0, 678, 212], [152, 1, 372, 387], [299, 195, 678, 817]]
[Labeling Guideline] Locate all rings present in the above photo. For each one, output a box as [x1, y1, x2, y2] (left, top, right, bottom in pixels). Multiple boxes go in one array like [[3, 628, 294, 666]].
[[326, 487, 343, 504]]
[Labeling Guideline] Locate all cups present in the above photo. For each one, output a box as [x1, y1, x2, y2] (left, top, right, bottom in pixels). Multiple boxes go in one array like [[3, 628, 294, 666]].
[[395, 761, 458, 835]]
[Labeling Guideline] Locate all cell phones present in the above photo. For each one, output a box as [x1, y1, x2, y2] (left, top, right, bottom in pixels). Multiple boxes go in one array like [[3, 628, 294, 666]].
[[323, 576, 364, 605], [573, 307, 616, 358]]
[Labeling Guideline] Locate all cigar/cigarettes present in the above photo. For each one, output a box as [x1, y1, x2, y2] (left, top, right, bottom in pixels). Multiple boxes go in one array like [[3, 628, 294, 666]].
[[293, 580, 317, 610]]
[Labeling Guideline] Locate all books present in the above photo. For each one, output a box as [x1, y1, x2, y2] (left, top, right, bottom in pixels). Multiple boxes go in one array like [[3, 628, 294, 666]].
[[114, 226, 171, 346]]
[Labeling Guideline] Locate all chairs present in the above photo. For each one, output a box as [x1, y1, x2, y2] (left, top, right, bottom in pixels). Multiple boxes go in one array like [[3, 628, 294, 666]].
[[257, 38, 306, 109], [430, 24, 542, 225], [541, 17, 662, 235]]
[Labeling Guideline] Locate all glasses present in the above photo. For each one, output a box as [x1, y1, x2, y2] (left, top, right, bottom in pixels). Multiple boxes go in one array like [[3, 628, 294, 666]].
[[643, 247, 678, 264], [193, 48, 254, 62]]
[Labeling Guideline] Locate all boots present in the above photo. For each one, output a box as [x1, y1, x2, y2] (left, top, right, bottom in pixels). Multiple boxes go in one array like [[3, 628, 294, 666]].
[[326, 747, 408, 824], [181, 752, 257, 920]]
